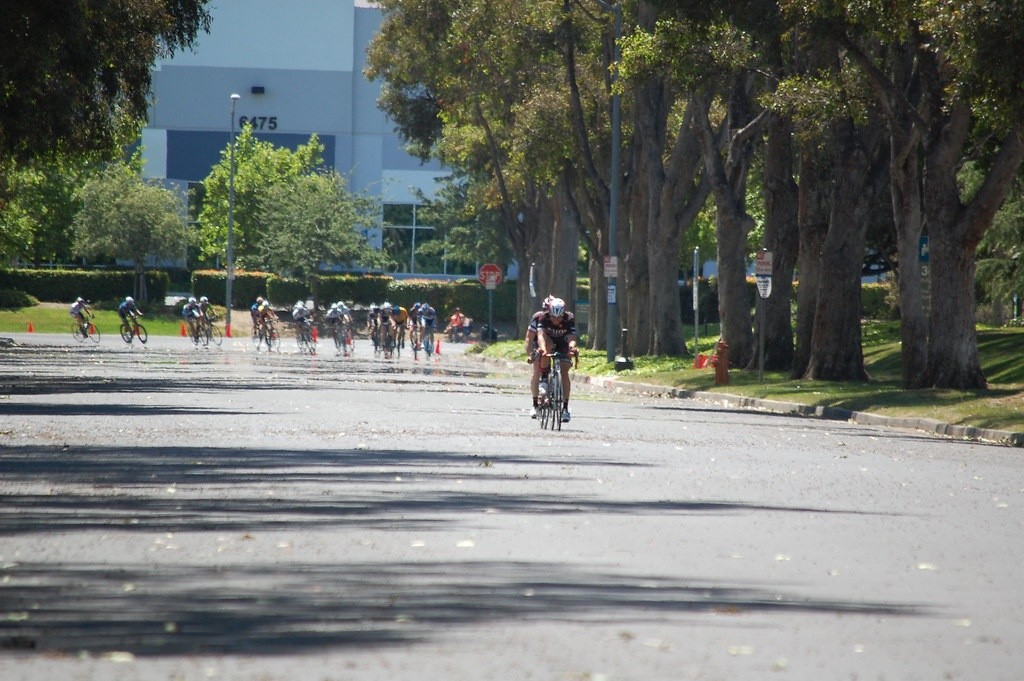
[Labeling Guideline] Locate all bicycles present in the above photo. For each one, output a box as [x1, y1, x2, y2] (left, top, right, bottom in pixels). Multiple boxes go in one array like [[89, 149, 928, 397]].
[[120, 315, 148, 344], [331, 321, 355, 357], [367, 324, 431, 361], [528, 349, 579, 432], [295, 321, 316, 355], [186, 316, 222, 347], [250, 318, 280, 350], [70, 314, 100, 344]]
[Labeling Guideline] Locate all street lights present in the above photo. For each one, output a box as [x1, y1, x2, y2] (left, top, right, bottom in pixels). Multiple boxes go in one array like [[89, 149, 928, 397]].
[[225, 94, 241, 323]]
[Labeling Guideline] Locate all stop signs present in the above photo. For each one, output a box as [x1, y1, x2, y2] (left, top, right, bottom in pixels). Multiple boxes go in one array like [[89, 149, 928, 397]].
[[479, 264, 502, 287]]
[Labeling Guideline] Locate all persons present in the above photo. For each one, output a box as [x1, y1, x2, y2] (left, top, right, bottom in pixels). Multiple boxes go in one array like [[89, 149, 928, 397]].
[[526, 295, 581, 422], [252, 297, 465, 351], [118, 296, 144, 334], [183, 296, 217, 343], [70, 297, 93, 338]]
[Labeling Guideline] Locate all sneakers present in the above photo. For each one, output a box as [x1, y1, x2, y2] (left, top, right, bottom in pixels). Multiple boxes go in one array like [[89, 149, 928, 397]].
[[125, 332, 131, 339], [402, 340, 404, 348], [531, 407, 537, 419], [562, 409, 570, 422], [539, 383, 547, 396], [430, 343, 434, 350], [413, 343, 417, 348]]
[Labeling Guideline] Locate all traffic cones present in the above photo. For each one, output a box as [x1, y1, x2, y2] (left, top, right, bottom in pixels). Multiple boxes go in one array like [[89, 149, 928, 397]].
[[180, 324, 186, 337], [29, 322, 33, 333], [89, 323, 96, 334], [434, 340, 440, 355], [224, 324, 231, 337]]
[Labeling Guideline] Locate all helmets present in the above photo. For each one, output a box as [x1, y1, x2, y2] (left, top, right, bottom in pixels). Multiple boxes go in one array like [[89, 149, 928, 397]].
[[393, 306, 399, 315], [76, 297, 83, 301], [338, 301, 343, 306], [256, 297, 262, 303], [414, 302, 421, 310], [263, 301, 270, 308], [422, 303, 429, 314], [373, 306, 379, 312], [331, 303, 338, 308], [200, 296, 208, 302], [126, 296, 134, 303], [188, 297, 196, 304], [383, 302, 391, 314], [295, 303, 303, 310], [542, 295, 565, 318]]
